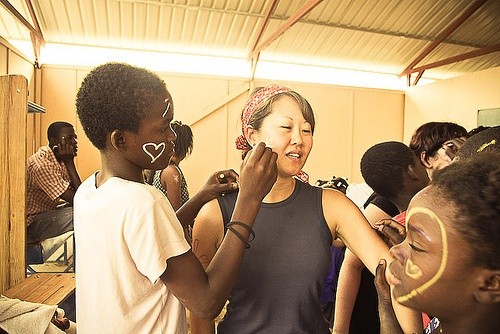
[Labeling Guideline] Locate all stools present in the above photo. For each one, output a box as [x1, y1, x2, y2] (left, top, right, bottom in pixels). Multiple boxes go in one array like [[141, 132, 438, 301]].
[[26, 230, 76, 279], [1, 272, 76, 323]]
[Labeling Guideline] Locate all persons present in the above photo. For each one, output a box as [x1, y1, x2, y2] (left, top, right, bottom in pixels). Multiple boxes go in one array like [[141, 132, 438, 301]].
[[331, 141, 430, 334], [72, 62, 280, 334], [374, 163, 500, 334], [441, 125, 500, 166], [189, 83, 424, 334], [24, 121, 83, 243], [409, 122, 470, 183], [144, 118, 193, 247]]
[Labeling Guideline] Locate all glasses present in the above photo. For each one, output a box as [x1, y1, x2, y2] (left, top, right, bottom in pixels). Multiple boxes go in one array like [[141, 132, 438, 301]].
[[424, 142, 458, 166]]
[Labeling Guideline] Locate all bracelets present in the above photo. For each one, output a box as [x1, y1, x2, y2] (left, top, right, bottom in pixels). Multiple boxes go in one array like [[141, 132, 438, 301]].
[[225, 221, 256, 242], [225, 226, 251, 250]]
